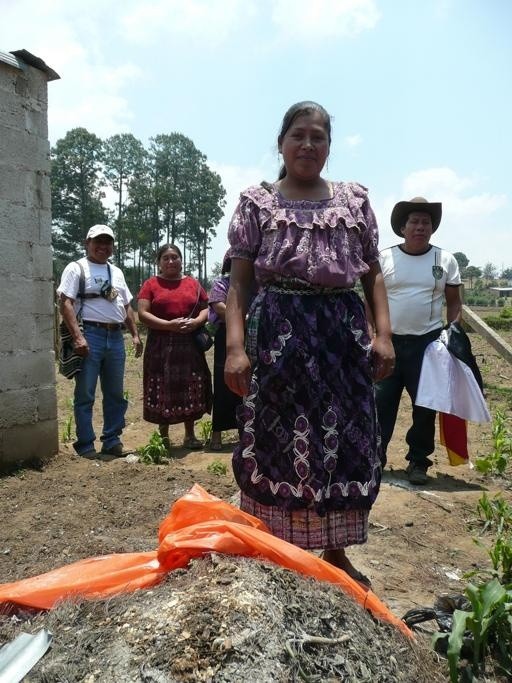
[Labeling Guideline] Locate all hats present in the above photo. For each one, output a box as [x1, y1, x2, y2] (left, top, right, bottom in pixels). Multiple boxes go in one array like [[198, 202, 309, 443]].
[[391, 198, 442, 238], [86, 225, 115, 241]]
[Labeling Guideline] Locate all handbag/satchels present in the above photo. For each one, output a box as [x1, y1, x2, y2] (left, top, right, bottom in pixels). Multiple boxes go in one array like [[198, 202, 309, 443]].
[[58, 260, 85, 379], [186, 283, 213, 352], [74, 259, 119, 301]]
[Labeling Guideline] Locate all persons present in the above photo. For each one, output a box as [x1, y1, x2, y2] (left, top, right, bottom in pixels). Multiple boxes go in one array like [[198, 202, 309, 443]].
[[56, 224, 143, 459], [224, 100, 396, 588], [365, 197, 463, 484], [137, 243, 210, 449], [207, 247, 243, 451]]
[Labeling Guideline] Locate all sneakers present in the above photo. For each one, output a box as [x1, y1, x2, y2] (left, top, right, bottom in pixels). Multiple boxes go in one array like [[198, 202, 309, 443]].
[[101, 445, 133, 457], [323, 556, 371, 589], [163, 437, 170, 449], [183, 439, 203, 448], [407, 457, 433, 484], [80, 450, 98, 460], [210, 439, 222, 451]]
